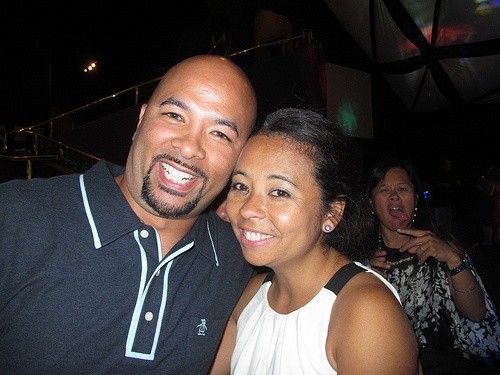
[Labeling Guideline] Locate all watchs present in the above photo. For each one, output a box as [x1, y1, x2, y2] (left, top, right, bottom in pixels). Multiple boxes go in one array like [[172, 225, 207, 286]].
[[449, 255, 471, 276]]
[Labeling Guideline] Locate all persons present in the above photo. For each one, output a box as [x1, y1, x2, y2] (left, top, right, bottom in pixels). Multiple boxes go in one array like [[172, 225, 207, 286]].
[[0, 55, 269, 375], [209, 108, 424, 375], [367, 158, 500, 375]]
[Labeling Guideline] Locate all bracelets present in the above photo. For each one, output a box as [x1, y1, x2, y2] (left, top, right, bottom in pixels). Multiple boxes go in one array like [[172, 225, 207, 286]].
[[449, 275, 477, 295]]
